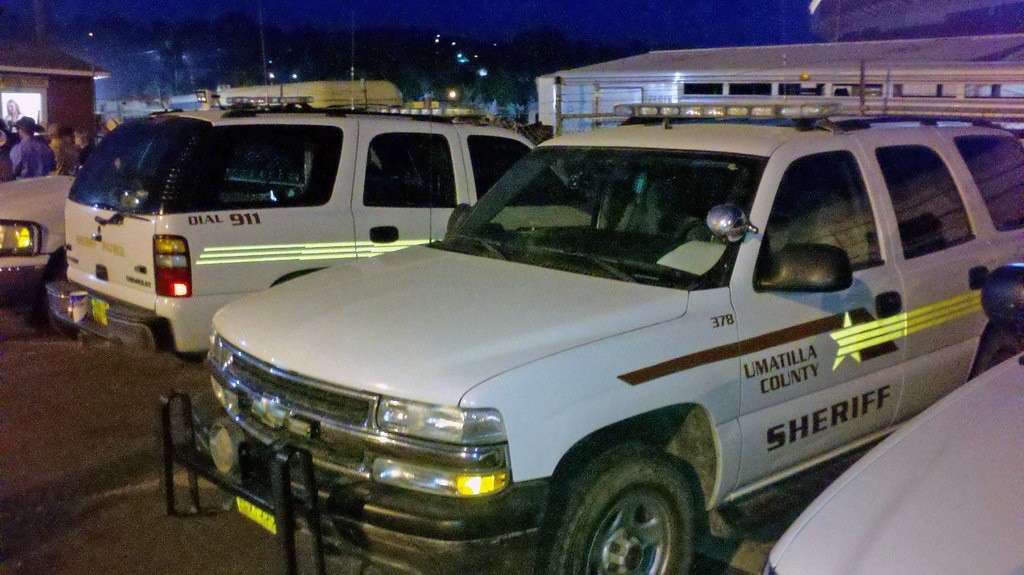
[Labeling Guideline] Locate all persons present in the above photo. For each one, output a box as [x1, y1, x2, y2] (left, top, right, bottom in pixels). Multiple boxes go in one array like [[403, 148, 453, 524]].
[[0, 116, 96, 183], [7, 100, 30, 133]]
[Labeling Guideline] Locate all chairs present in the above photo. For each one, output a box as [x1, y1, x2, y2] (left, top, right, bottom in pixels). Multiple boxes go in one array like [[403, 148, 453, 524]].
[[618, 164, 705, 242]]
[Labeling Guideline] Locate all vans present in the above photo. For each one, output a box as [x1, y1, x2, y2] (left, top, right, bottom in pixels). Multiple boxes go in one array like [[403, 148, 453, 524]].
[[44, 80, 602, 352]]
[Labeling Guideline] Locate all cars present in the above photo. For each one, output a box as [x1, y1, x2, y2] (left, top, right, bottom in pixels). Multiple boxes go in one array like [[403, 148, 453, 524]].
[[158, 103, 1024, 575], [0, 176, 101, 285]]
